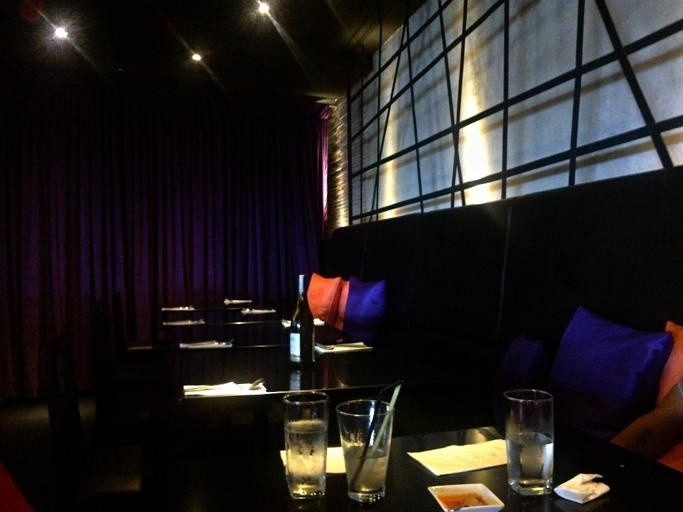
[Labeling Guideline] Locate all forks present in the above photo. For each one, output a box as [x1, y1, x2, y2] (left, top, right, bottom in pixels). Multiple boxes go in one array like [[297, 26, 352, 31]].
[[316, 342, 374, 350]]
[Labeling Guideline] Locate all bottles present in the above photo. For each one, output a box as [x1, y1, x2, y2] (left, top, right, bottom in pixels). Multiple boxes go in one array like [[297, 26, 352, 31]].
[[289, 273, 315, 372]]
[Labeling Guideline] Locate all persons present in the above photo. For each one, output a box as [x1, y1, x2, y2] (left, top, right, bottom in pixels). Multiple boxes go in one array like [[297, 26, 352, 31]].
[[610, 379, 683, 460]]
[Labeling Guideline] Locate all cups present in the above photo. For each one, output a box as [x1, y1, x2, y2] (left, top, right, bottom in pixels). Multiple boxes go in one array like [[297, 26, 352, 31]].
[[334, 399, 394, 502], [503, 390, 558, 496], [282, 391, 331, 501]]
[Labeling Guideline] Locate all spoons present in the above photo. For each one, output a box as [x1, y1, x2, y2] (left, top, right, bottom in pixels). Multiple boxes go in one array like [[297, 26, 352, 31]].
[[449, 497, 478, 512]]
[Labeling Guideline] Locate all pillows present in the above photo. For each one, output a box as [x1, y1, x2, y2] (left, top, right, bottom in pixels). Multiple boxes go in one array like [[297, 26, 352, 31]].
[[544, 305, 672, 427], [491, 334, 554, 419], [332, 279, 349, 330], [308, 272, 345, 336], [345, 276, 387, 334], [654, 319, 683, 468]]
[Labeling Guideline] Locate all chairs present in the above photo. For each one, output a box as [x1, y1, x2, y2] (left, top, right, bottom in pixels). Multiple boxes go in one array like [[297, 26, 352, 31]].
[[113, 291, 167, 370], [45, 338, 147, 511], [90, 313, 162, 441]]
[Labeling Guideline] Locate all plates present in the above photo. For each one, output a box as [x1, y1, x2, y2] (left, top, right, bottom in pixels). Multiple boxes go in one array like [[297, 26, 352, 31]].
[[427, 483, 505, 512]]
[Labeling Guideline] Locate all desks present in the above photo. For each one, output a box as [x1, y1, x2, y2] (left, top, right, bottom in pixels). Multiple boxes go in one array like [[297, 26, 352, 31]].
[[161, 305, 459, 410], [272, 415, 683, 512]]
